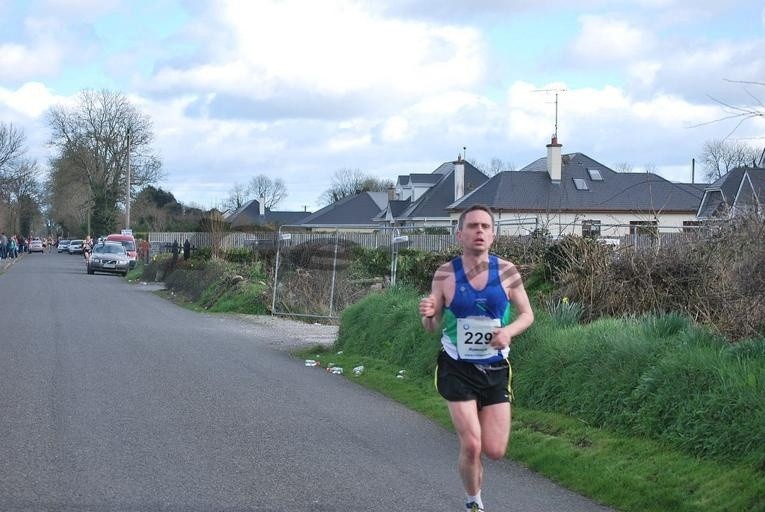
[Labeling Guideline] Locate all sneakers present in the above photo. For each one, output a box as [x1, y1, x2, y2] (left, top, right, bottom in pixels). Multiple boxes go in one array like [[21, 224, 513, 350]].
[[464, 501, 485, 512]]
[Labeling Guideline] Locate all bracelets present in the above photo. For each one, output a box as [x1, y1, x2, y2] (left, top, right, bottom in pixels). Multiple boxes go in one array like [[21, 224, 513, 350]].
[[424, 315, 435, 318]]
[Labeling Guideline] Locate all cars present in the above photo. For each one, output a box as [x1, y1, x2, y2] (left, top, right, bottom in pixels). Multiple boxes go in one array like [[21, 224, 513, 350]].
[[28, 241, 44, 254], [55, 230, 139, 277]]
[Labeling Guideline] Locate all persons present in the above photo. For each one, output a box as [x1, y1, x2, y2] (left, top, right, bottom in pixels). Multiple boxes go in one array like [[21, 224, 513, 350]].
[[0, 232, 62, 260], [82, 236, 98, 259], [418, 203, 535, 512]]
